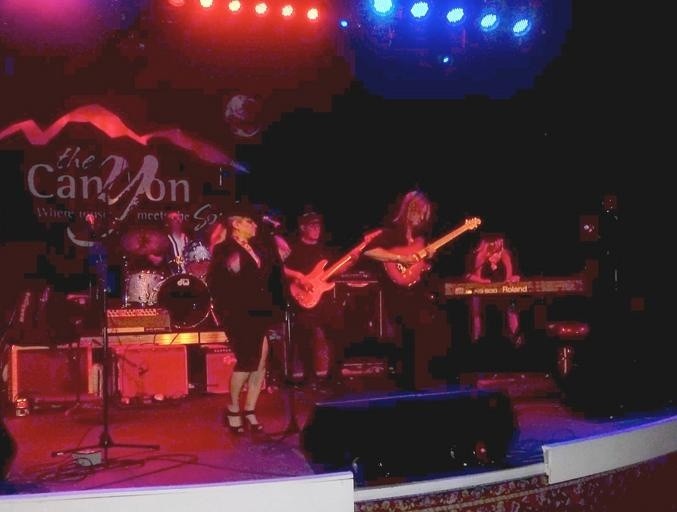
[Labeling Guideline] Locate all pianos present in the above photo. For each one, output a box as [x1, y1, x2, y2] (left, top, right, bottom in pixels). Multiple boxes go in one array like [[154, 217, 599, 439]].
[[436, 276, 592, 298]]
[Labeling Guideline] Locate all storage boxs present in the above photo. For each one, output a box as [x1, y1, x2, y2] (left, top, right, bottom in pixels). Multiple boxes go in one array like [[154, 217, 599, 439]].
[[5, 341, 102, 416]]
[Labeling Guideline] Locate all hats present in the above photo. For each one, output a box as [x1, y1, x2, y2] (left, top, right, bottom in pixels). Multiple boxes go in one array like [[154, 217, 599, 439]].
[[298, 211, 323, 225]]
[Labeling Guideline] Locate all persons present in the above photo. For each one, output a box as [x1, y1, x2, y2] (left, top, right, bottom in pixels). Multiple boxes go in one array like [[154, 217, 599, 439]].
[[360, 190, 452, 394], [464, 233, 526, 353], [161, 211, 199, 276], [280, 210, 346, 387], [206, 208, 274, 436]]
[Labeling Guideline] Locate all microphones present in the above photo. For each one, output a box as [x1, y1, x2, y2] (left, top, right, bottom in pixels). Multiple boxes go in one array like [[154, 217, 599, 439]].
[[84, 214, 97, 236]]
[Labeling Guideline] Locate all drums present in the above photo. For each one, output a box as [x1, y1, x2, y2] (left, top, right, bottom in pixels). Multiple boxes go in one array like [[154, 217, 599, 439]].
[[121, 269, 167, 306], [152, 274, 211, 329], [183, 240, 212, 278]]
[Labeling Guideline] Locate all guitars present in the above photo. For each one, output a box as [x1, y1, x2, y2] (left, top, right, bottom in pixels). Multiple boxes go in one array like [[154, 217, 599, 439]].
[[290, 230, 382, 308], [384, 217, 481, 287]]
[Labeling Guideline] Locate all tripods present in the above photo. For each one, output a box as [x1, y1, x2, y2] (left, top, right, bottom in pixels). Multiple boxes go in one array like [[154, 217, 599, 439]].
[[50, 222, 160, 469]]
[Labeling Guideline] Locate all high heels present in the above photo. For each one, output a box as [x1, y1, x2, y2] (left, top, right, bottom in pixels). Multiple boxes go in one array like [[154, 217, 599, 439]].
[[223, 407, 244, 433], [242, 409, 263, 432]]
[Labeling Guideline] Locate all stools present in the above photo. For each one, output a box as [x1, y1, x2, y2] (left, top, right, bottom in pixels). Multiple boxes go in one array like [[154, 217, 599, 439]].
[[550, 321, 589, 406]]
